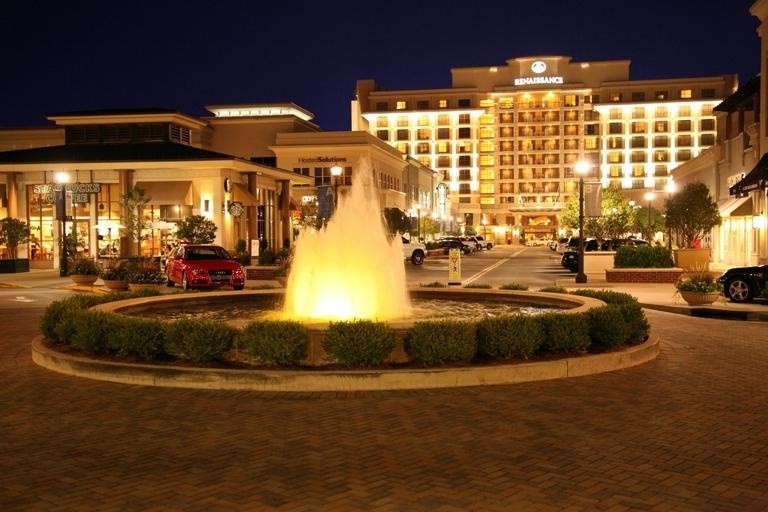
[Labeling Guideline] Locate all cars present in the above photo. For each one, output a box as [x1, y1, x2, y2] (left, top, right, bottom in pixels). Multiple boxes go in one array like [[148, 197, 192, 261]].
[[428, 236, 498, 255], [715, 260, 768, 304], [164, 244, 249, 292], [527, 236, 655, 272]]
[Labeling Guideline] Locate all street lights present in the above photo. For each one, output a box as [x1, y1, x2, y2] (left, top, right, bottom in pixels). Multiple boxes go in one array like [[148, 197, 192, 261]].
[[414, 201, 423, 244], [330, 159, 342, 210], [573, 156, 592, 283], [52, 167, 71, 279]]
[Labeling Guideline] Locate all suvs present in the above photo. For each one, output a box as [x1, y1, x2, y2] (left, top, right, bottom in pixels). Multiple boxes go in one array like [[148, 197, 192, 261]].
[[387, 233, 429, 266]]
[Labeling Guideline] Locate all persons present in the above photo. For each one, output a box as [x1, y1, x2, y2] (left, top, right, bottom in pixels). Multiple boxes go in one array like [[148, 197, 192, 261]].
[[30, 234, 40, 260], [100, 245, 109, 255]]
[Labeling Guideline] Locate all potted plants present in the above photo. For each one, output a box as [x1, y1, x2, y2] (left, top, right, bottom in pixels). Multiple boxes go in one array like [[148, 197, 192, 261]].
[[66, 253, 169, 294], [0, 215, 34, 273], [662, 178, 723, 268], [674, 257, 725, 302]]
